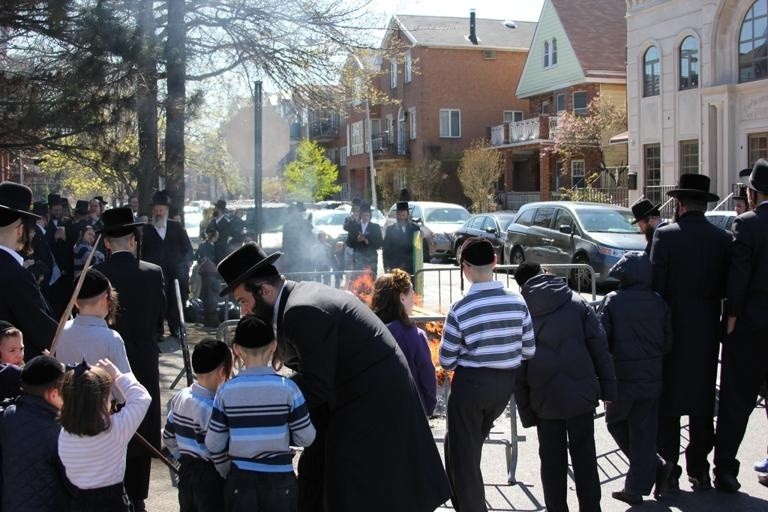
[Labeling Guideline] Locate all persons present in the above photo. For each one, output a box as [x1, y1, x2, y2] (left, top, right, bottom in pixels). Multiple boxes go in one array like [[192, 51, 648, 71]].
[[628, 198, 667, 254], [752, 456, 768, 487], [730, 181, 751, 215], [434, 236, 539, 511], [508, 261, 616, 511], [644, 172, 732, 497], [593, 250, 682, 506], [710, 155, 768, 493]]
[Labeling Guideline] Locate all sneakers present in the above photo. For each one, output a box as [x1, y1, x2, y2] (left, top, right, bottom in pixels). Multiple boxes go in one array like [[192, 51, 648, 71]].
[[753, 457, 768, 488], [610, 461, 742, 507]]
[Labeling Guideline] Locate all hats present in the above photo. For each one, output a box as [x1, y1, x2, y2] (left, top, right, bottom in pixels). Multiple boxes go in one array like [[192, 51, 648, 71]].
[[630, 199, 662, 225], [216, 241, 283, 298], [739, 158, 768, 194], [213, 200, 229, 212], [460, 236, 495, 266], [71, 268, 110, 300], [96, 207, 142, 237], [731, 183, 748, 200], [19, 355, 66, 386], [191, 338, 228, 374], [234, 314, 275, 349], [31, 193, 193, 216], [392, 201, 412, 211], [349, 197, 374, 213], [0, 181, 42, 221], [666, 173, 720, 203]]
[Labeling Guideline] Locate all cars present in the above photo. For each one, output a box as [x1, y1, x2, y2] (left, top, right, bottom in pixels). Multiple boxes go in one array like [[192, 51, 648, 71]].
[[704, 210, 737, 231]]
